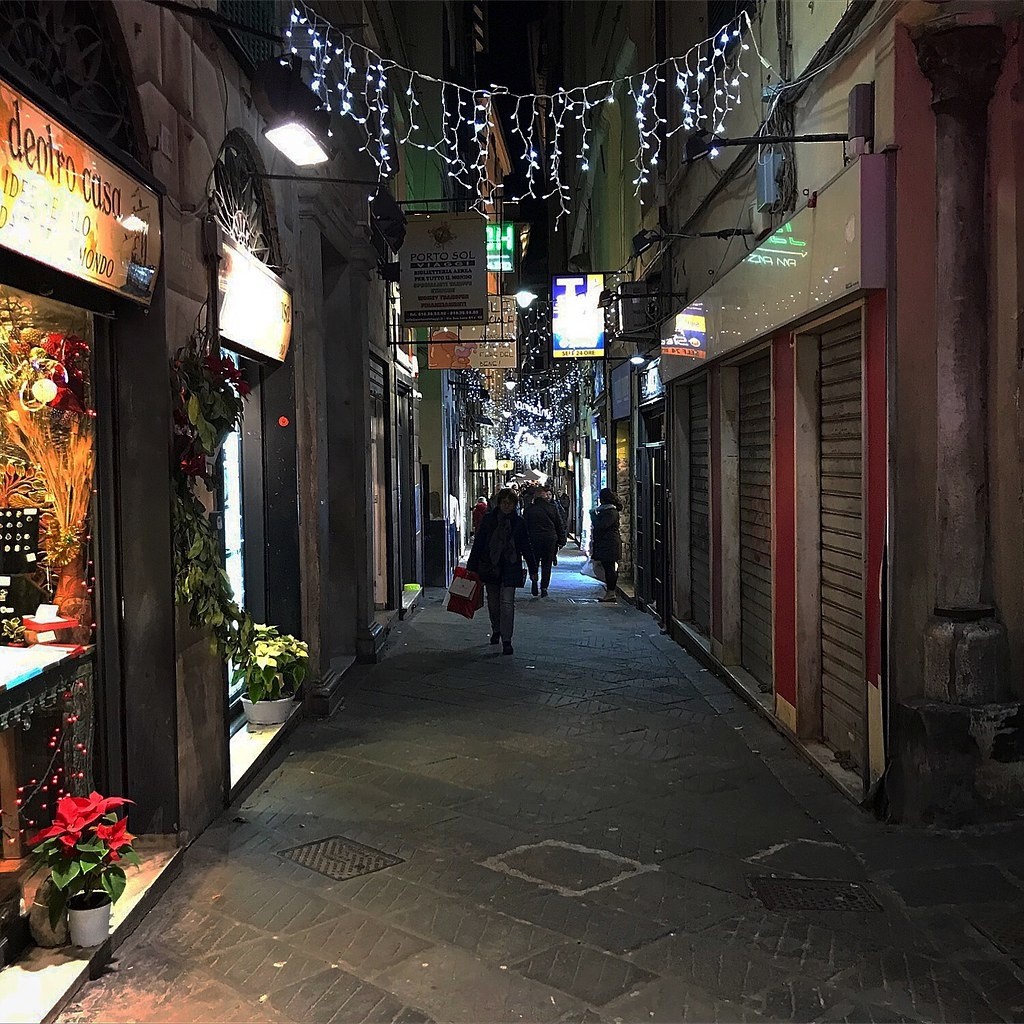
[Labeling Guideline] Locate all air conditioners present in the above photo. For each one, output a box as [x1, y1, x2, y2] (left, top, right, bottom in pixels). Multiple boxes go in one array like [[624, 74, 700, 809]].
[[616, 281, 649, 332]]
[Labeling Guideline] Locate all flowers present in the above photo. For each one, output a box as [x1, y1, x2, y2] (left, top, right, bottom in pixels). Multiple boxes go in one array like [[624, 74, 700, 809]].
[[25, 791, 140, 935], [170, 335, 255, 659]]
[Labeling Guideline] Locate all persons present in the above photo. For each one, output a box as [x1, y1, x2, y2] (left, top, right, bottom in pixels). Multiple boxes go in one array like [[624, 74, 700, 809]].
[[524, 490, 567, 597], [492, 480, 567, 566], [466, 488, 538, 654], [473, 497, 487, 531], [589, 488, 623, 602], [450, 494, 460, 551]]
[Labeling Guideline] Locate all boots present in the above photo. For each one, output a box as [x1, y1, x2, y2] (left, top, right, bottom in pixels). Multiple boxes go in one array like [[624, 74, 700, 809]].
[[598, 590, 616, 602]]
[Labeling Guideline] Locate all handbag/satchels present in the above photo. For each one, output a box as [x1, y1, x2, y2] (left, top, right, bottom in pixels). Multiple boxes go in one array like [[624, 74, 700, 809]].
[[446, 567, 484, 619], [580, 557, 605, 582]]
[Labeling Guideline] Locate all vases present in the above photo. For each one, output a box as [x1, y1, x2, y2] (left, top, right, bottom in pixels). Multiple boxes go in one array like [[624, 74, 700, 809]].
[[236, 690, 295, 726], [65, 892, 111, 949]]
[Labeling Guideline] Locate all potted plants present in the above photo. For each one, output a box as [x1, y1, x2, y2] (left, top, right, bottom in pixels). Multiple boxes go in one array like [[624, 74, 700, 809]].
[[230, 623, 309, 703]]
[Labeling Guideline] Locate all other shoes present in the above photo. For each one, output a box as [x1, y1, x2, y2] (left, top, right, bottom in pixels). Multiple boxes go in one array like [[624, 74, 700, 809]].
[[532, 582, 538, 596], [491, 632, 500, 645], [540, 588, 548, 599], [553, 557, 557, 566], [503, 641, 513, 655]]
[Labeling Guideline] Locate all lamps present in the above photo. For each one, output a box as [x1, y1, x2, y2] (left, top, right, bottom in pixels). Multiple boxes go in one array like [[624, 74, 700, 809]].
[[449, 380, 490, 402], [631, 229, 755, 259], [462, 413, 493, 427], [502, 377, 518, 391], [597, 288, 684, 309], [252, 173, 408, 256], [680, 130, 849, 166], [143, 0, 332, 167]]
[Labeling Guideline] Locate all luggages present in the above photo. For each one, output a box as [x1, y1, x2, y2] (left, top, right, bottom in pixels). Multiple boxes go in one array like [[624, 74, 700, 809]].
[[522, 561, 527, 585]]
